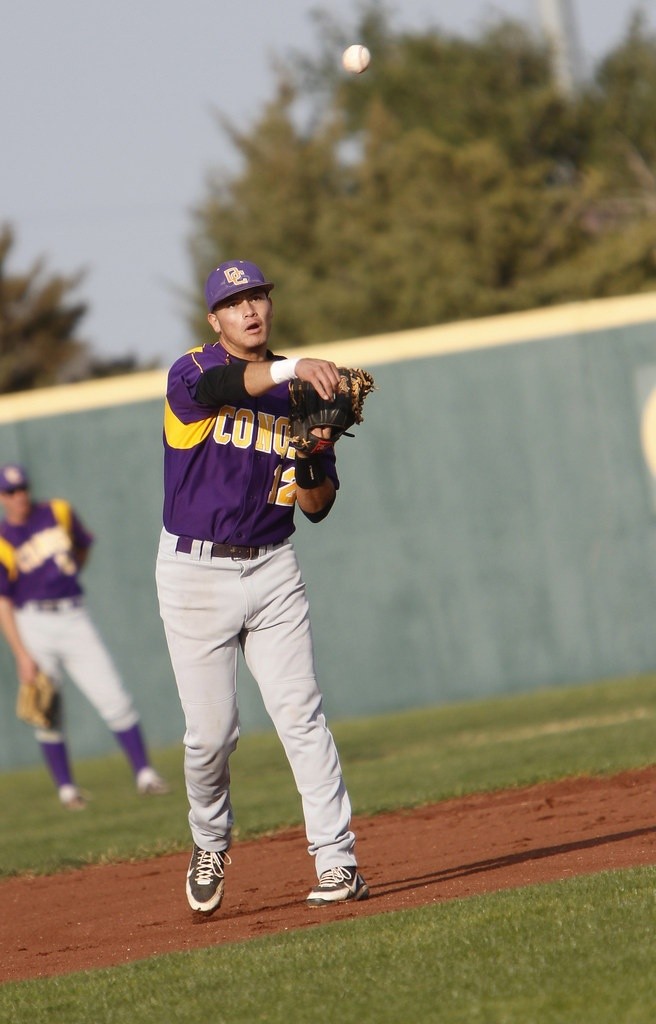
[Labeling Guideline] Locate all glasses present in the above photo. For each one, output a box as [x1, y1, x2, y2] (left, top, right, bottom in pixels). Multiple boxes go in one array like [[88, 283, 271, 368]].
[[6, 484, 26, 492]]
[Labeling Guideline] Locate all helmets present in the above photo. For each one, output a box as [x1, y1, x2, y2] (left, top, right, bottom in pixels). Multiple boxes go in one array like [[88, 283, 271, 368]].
[[205, 260, 274, 313]]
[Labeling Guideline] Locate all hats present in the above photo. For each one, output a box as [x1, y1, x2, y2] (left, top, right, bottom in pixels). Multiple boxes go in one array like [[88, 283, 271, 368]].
[[0, 466, 25, 490]]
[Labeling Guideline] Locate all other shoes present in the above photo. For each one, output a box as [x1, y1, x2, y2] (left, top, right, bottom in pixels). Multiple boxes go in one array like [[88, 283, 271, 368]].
[[61, 794, 88, 813], [137, 775, 171, 795]]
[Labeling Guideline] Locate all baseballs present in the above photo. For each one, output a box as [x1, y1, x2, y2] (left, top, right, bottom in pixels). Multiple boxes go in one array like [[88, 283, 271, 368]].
[[342, 44, 371, 75]]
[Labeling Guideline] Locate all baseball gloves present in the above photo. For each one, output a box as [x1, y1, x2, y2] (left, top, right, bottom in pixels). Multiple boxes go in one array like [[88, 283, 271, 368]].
[[15, 674, 63, 730], [289, 367, 374, 455]]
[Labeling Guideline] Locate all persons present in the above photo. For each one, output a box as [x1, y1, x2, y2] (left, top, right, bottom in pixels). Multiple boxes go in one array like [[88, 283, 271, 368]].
[[156, 260, 376, 913], [0, 462, 173, 812]]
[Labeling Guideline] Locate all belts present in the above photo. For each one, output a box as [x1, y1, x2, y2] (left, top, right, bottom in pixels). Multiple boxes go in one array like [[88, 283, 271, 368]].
[[176, 536, 283, 561], [38, 599, 80, 610]]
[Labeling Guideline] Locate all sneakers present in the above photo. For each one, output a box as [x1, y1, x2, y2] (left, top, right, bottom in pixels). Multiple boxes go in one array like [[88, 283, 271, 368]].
[[306, 867, 370, 909], [185, 843, 231, 914]]
